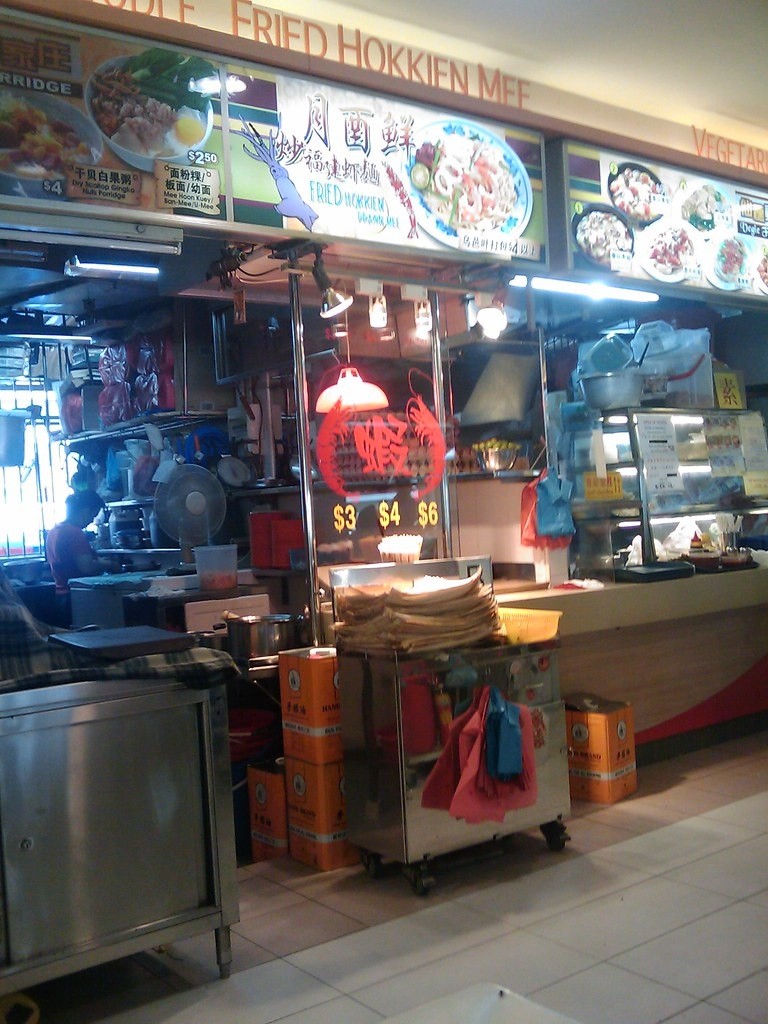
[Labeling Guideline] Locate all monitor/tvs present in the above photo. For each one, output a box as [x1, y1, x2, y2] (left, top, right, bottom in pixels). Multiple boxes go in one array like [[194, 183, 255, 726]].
[[213, 300, 339, 383]]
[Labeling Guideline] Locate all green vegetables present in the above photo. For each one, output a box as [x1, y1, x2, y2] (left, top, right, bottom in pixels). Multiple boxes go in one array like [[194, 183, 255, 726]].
[[120, 47, 217, 110]]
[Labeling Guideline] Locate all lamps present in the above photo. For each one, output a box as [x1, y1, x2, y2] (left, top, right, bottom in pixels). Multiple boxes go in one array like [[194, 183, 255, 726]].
[[414, 300, 431, 332], [368, 296, 387, 329], [0, 210, 184, 256], [476, 283, 511, 338], [311, 247, 353, 319]]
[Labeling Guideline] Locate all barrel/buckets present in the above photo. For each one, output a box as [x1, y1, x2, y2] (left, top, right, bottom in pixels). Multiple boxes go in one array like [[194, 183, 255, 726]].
[[190, 543, 237, 590], [248, 511, 305, 569], [228, 709, 277, 858]]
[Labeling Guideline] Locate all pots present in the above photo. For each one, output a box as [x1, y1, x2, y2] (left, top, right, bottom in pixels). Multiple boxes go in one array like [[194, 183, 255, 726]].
[[3, 558, 46, 583], [224, 614, 303, 663]]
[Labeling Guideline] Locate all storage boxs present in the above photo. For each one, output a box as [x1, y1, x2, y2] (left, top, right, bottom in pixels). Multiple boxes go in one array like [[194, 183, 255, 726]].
[[565, 690, 639, 805], [284, 755, 353, 873], [247, 759, 290, 864], [278, 646, 344, 766], [185, 594, 271, 634]]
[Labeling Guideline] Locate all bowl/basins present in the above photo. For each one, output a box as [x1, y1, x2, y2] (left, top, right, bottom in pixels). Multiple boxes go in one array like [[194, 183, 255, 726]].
[[578, 369, 644, 409], [572, 162, 668, 272], [475, 448, 519, 473], [0, 54, 213, 202]]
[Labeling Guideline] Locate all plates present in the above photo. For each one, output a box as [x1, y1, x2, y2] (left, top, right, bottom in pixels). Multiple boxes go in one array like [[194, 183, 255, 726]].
[[637, 185, 768, 297], [402, 118, 534, 251]]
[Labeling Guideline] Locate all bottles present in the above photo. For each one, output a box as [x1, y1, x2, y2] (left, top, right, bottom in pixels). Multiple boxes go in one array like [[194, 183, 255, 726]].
[[434, 683, 454, 747], [690, 531, 702, 549]]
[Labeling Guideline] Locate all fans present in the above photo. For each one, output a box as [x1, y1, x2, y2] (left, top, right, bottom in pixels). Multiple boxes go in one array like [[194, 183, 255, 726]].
[[155, 463, 227, 547]]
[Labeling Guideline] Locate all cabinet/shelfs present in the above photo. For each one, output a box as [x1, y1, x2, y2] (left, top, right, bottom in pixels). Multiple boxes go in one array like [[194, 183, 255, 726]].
[[65, 410, 543, 507], [568, 405, 768, 584]]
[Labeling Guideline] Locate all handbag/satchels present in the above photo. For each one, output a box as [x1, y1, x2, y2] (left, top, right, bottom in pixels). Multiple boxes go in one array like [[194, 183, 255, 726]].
[[420, 678, 538, 824]]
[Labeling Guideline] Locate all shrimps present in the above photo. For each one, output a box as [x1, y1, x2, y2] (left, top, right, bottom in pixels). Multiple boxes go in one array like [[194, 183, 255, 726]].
[[317, 397, 360, 500], [406, 366, 445, 501]]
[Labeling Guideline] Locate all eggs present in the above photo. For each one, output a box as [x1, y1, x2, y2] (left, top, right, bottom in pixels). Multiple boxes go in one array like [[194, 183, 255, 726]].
[[163, 105, 207, 156]]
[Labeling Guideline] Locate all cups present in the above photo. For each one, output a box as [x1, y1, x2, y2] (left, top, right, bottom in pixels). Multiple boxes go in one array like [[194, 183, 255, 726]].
[[720, 532, 737, 552]]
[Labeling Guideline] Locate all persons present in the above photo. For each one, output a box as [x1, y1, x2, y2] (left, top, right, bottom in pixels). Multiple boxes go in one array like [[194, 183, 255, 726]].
[[44, 489, 121, 621]]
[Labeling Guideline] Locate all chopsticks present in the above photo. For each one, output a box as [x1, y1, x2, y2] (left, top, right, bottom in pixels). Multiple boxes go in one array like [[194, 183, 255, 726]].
[[378, 534, 424, 563], [715, 512, 744, 532]]
